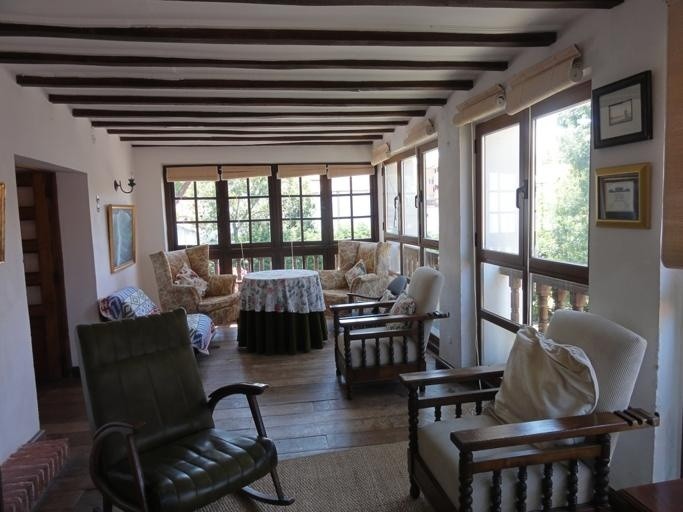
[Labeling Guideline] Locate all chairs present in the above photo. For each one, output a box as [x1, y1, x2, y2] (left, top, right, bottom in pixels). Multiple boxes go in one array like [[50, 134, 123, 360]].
[[329, 266, 445, 400], [77, 306, 295, 512], [149, 244, 240, 325], [399, 309, 659, 512], [318, 240, 392, 317], [347, 275, 407, 315], [100, 287, 217, 356]]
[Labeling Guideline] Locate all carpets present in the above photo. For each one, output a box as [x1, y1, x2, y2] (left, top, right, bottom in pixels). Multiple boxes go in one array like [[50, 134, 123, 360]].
[[75, 440, 434, 512]]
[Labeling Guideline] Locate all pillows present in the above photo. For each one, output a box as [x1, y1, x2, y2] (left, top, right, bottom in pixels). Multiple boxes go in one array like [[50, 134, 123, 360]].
[[485, 324, 599, 472], [386, 293, 415, 329], [174, 262, 209, 298], [345, 259, 367, 290]]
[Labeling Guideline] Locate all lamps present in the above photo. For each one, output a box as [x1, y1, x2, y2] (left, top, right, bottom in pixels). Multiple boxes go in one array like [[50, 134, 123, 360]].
[[114, 174, 136, 194]]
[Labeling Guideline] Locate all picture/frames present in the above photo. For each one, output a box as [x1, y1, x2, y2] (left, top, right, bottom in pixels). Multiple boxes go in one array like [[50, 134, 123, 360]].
[[109, 205, 136, 273], [595, 161, 652, 229], [591, 69, 653, 148]]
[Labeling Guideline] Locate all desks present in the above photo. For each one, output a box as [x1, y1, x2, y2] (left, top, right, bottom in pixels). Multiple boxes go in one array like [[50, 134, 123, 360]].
[[237, 269, 328, 355]]
